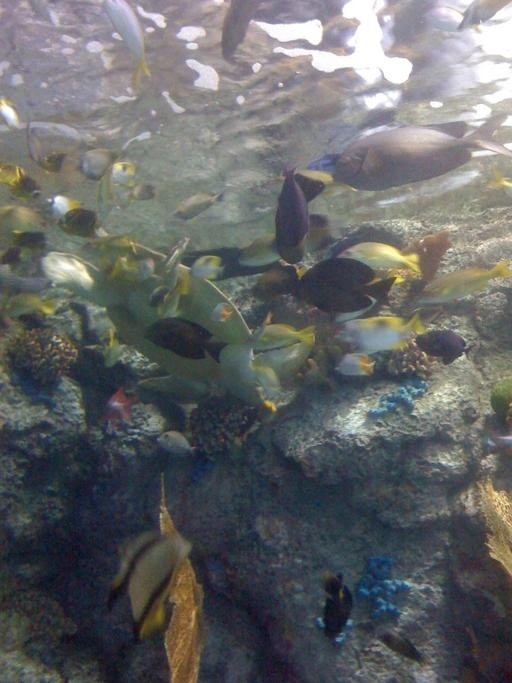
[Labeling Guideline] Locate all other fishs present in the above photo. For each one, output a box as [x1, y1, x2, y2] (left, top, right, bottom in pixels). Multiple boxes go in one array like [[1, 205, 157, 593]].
[[1, 100, 511, 425]]
